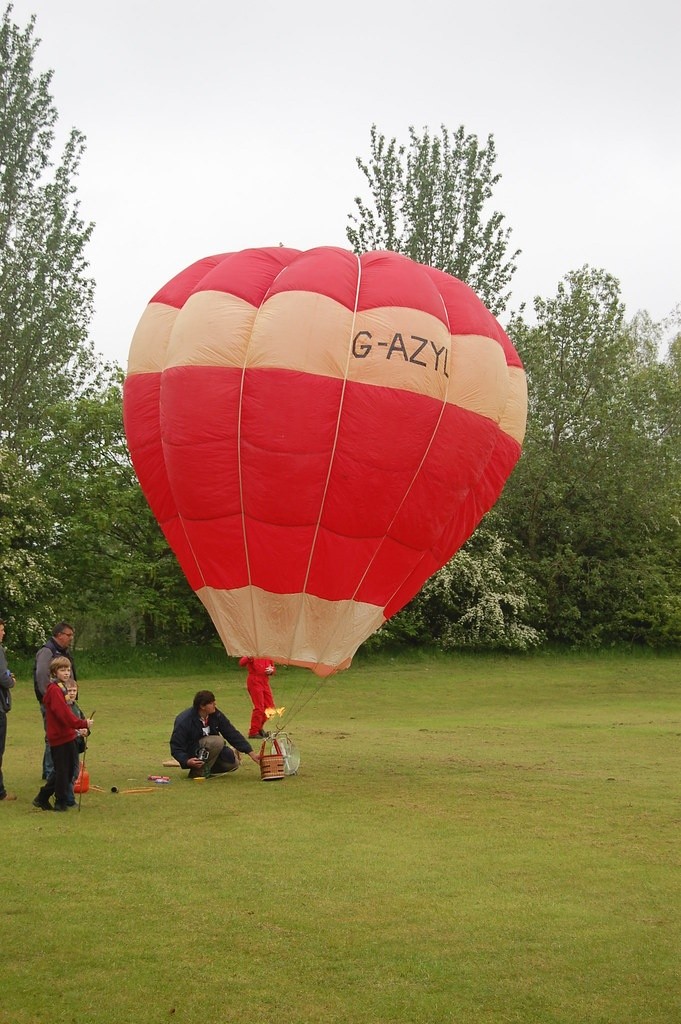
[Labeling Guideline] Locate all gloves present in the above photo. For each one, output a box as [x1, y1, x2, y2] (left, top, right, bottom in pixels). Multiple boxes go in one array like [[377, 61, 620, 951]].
[[264, 665, 274, 675]]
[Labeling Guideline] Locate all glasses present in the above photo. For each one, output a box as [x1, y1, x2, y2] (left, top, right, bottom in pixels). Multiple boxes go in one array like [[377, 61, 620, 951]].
[[59, 631, 77, 638]]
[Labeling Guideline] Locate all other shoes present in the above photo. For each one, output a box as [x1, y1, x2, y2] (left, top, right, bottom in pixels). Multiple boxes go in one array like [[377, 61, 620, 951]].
[[42, 771, 52, 780]]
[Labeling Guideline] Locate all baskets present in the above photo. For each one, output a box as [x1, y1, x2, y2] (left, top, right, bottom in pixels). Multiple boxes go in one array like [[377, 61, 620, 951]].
[[258, 738, 286, 780]]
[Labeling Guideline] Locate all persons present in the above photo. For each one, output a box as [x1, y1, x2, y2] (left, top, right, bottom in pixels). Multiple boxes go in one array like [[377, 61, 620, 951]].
[[241, 657, 274, 739], [0, 621, 94, 812], [171, 690, 261, 779]]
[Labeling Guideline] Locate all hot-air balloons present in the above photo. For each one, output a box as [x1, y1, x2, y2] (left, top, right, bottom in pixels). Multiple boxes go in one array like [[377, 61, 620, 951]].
[[122, 245, 528, 782]]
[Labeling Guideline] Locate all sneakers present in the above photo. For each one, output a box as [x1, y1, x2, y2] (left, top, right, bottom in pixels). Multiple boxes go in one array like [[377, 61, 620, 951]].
[[3, 793, 19, 802], [187, 771, 212, 779], [32, 797, 81, 813], [247, 729, 270, 739]]
